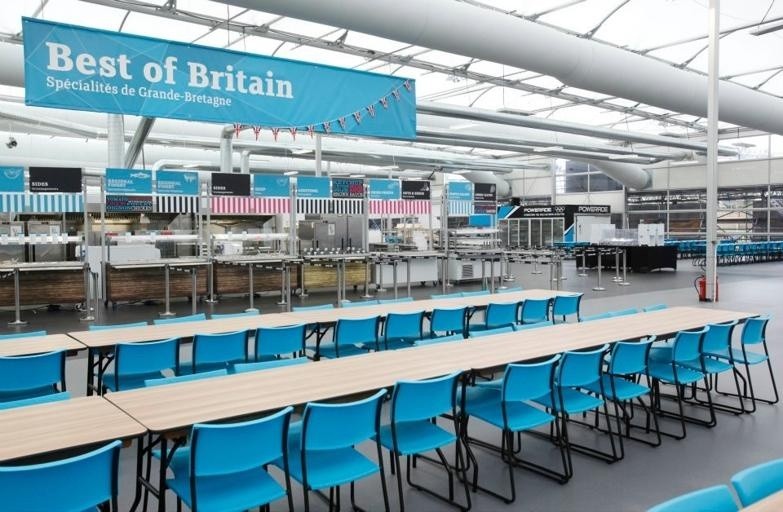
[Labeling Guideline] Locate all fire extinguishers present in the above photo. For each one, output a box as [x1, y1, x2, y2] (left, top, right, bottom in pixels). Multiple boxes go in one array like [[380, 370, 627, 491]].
[[694, 275, 718, 301]]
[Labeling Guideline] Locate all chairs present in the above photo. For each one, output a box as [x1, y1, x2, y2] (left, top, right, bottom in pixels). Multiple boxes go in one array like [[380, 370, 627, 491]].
[[554, 240, 783, 266], [0, 286, 783, 512]]
